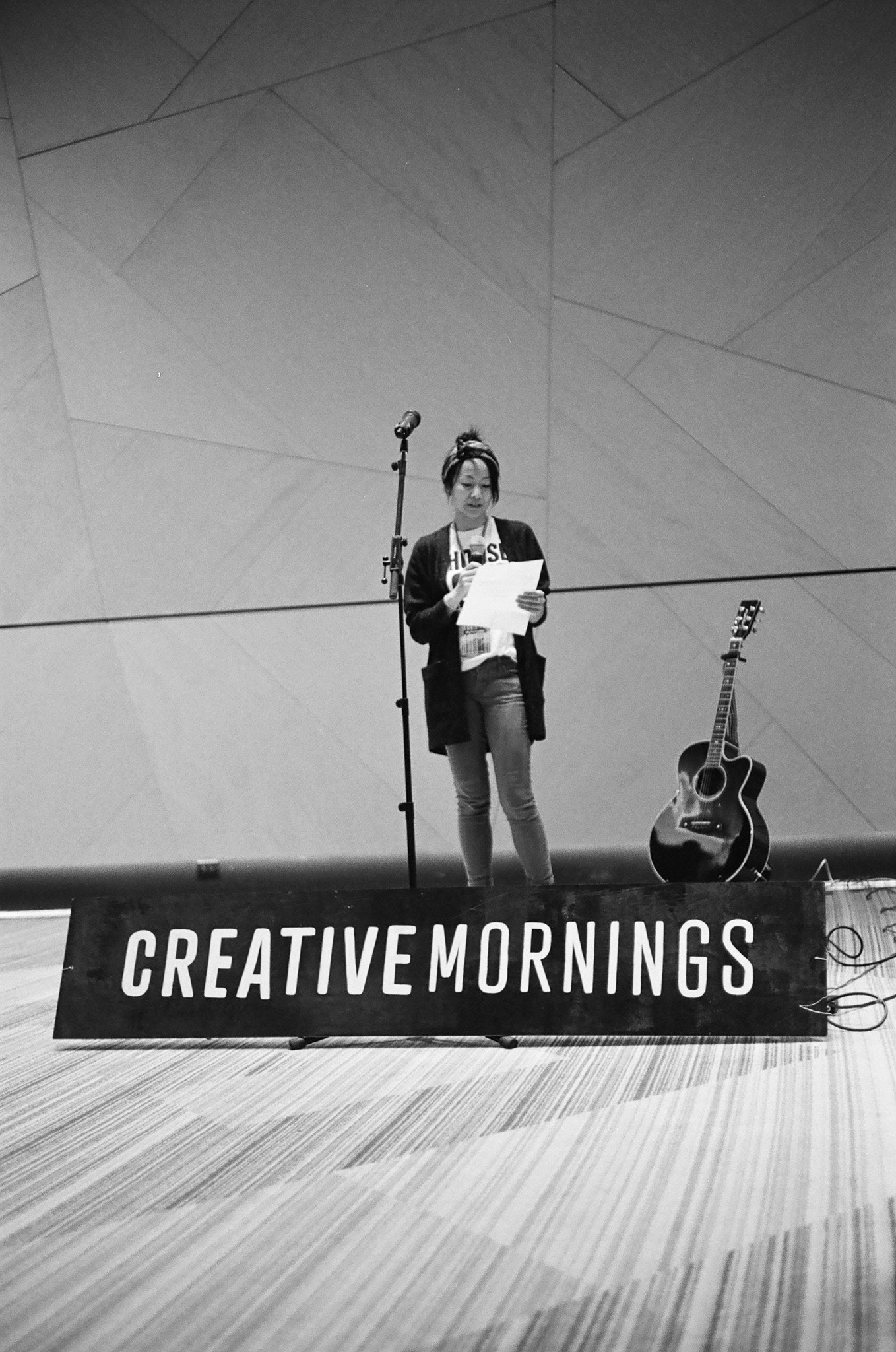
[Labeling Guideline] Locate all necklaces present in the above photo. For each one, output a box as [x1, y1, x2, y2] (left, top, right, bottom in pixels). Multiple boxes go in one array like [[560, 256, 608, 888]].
[[452, 516, 489, 560]]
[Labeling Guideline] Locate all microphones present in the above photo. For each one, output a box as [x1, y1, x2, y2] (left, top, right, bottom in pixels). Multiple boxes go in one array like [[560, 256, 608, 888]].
[[394, 410, 421, 438]]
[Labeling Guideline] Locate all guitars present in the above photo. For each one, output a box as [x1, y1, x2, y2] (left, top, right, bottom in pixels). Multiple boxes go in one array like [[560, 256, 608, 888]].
[[647, 599, 772, 883]]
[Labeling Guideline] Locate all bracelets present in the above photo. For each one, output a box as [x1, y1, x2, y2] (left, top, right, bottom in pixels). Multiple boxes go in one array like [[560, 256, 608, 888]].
[[443, 595, 460, 611]]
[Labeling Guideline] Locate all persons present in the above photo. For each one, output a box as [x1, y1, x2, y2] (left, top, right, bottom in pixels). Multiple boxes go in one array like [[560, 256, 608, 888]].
[[399, 424, 558, 885]]
